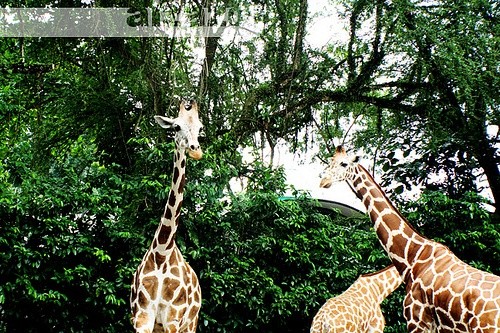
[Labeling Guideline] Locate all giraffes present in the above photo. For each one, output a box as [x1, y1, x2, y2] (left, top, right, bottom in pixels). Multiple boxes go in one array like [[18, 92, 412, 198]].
[[130, 98, 204, 333], [320, 144, 500, 333], [310, 264, 402, 333]]
